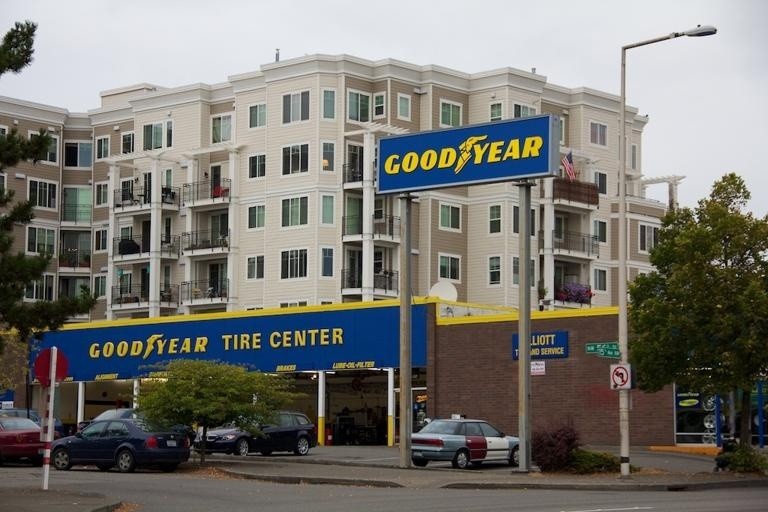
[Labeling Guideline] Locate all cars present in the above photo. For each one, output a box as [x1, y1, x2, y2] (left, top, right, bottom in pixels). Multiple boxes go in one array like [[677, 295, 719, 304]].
[[410, 413, 519, 469], [193, 409, 318, 455], [50, 418, 191, 473], [90, 407, 138, 422], [0, 415, 44, 466]]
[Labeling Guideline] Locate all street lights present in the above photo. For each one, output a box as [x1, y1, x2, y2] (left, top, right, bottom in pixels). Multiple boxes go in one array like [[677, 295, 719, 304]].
[[618, 25, 718, 478]]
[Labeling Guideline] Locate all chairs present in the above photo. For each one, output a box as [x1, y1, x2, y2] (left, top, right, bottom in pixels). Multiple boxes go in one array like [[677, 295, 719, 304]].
[[128, 193, 138, 205]]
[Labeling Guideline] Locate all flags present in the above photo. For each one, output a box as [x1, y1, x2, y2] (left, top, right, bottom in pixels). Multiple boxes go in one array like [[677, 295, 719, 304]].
[[561, 149, 577, 184]]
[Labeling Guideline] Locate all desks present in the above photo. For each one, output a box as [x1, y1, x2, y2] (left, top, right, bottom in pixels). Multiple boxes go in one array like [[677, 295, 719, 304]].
[[137, 195, 144, 205]]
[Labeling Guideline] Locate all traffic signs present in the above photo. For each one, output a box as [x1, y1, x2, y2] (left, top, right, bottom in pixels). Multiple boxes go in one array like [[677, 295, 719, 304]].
[[597, 348, 621, 358], [585, 343, 619, 354]]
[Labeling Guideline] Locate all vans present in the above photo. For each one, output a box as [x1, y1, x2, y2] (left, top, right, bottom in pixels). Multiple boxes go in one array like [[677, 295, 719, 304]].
[[0, 408, 40, 427]]
